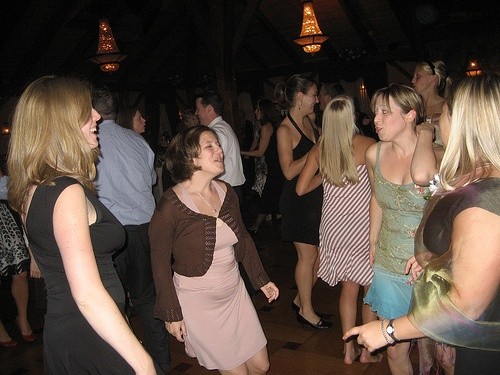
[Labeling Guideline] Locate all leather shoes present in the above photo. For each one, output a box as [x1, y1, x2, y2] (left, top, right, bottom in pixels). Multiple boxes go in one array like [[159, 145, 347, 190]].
[[251, 292, 273, 312]]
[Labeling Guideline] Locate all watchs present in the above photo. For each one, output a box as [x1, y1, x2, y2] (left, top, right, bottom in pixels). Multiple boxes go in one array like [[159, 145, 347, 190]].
[[386, 319, 401, 342]]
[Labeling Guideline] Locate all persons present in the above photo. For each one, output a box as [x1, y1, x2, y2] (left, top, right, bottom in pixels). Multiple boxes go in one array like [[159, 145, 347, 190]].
[[1, 53, 482, 365], [146, 123, 279, 375], [8, 73, 161, 374], [339, 75, 500, 375]]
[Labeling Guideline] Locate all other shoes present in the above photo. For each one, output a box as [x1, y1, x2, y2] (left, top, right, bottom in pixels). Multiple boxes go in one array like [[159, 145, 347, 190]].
[[246, 224, 258, 234], [275, 218, 282, 224], [262, 219, 272, 225]]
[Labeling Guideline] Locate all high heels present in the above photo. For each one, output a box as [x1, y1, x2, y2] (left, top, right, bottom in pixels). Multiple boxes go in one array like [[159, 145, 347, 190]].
[[291, 301, 326, 319], [297, 313, 333, 329], [0, 339, 17, 349], [14, 322, 36, 342]]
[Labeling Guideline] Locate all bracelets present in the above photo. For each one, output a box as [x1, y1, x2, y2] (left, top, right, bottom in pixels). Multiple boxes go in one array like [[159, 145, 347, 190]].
[[381, 317, 395, 345]]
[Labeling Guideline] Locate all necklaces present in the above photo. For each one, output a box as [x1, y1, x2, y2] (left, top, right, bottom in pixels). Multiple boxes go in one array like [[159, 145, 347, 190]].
[[191, 181, 217, 213]]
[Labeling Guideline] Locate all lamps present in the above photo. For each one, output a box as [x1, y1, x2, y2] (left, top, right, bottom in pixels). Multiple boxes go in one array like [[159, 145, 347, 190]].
[[88, 0, 128, 72], [292, 0, 329, 56], [465, 56, 482, 76]]
